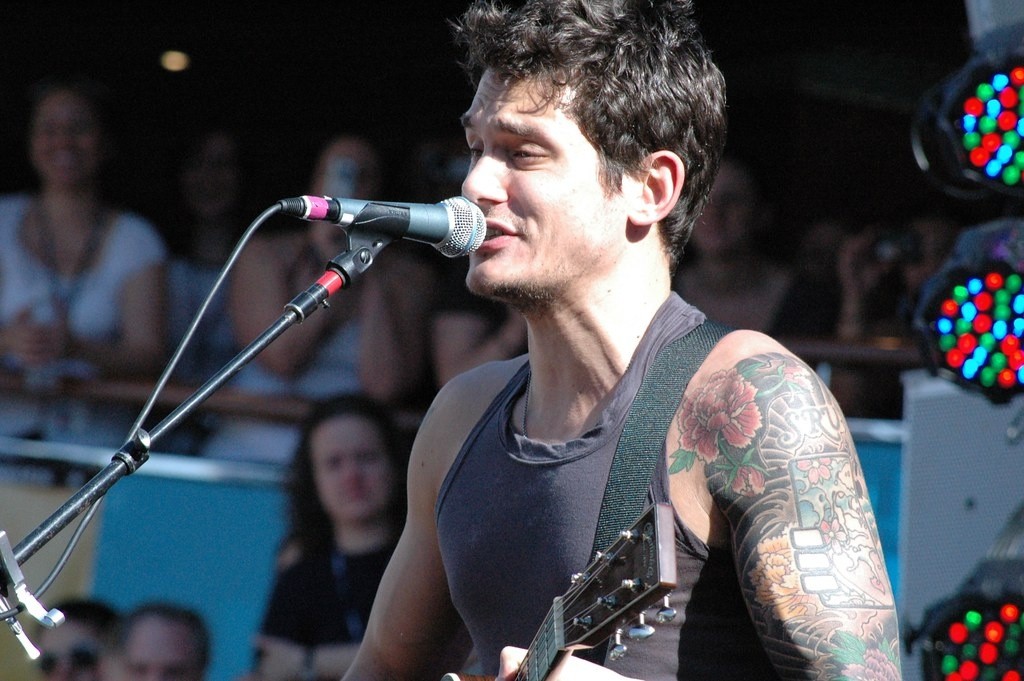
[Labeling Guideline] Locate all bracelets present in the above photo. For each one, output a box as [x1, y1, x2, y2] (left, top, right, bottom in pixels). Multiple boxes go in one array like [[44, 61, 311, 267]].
[[298, 644, 319, 681]]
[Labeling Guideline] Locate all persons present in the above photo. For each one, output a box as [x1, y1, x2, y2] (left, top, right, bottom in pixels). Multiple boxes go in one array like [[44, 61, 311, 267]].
[[28, 599, 120, 681], [335, 0, 912, 681], [97, 602, 211, 681], [0, 65, 965, 451], [245, 388, 417, 681]]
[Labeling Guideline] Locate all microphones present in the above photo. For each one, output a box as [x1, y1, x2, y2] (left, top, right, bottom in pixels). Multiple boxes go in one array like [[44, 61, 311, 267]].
[[294, 195, 487, 257]]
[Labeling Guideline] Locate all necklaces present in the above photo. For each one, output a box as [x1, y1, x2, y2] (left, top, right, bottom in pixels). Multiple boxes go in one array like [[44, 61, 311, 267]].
[[519, 369, 535, 439], [30, 193, 108, 322]]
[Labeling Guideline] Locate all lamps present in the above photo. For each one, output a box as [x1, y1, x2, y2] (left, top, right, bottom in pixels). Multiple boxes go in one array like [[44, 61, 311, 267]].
[[915, 220, 1023, 403], [911, 52, 1024, 207], [904, 556, 1024, 681]]
